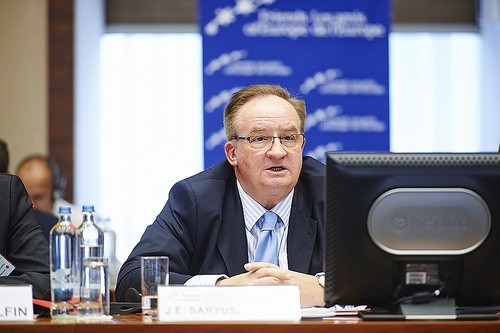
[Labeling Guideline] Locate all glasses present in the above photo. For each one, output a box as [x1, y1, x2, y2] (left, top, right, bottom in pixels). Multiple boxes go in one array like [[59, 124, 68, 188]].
[[231, 134, 305, 148]]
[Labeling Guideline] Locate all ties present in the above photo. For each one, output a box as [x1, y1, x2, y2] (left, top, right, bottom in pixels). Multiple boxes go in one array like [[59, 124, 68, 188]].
[[253, 211, 282, 263]]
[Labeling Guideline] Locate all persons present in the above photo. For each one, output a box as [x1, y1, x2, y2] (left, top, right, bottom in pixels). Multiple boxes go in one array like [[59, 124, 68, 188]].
[[113, 85, 327, 309], [0, 140, 82, 316]]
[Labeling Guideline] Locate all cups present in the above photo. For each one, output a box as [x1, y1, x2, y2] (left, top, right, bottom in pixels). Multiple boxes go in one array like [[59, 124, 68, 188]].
[[83, 258, 110, 320], [141, 257, 169, 314]]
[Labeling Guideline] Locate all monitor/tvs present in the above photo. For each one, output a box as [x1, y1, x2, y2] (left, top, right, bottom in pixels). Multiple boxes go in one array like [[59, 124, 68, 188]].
[[324, 151, 500, 320]]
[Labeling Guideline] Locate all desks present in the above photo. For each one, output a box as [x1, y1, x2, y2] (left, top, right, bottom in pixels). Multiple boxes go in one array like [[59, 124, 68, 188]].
[[0, 306, 500, 333]]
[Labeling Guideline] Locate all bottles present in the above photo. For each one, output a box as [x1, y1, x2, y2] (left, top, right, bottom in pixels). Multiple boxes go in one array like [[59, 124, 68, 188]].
[[49, 207, 81, 319], [78, 205, 104, 319]]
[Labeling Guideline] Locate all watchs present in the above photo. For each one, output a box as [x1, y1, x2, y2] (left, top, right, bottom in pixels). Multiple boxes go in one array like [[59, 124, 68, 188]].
[[315, 272, 325, 287]]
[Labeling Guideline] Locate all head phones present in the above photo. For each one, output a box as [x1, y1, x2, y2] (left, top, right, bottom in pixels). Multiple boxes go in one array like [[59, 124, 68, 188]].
[[48, 159, 67, 200]]
